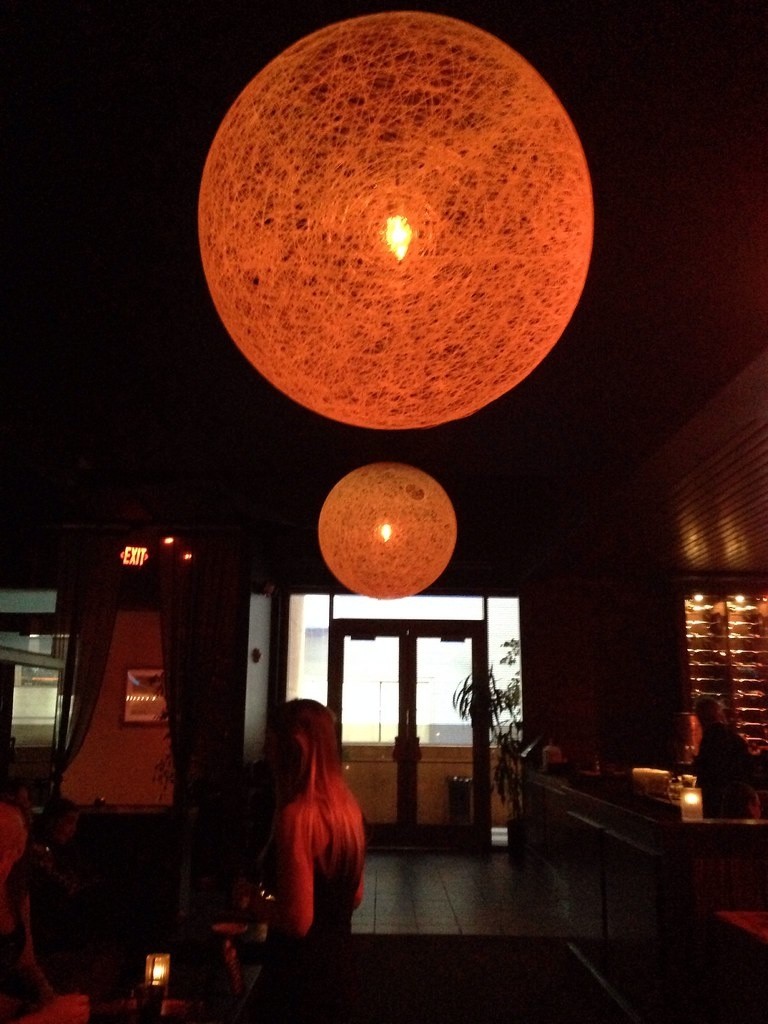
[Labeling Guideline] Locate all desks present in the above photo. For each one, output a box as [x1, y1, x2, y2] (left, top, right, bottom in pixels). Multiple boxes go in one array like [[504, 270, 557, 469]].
[[527, 768, 768, 1024]]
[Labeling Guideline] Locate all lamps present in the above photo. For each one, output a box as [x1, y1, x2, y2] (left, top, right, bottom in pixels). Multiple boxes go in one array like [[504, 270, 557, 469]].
[[199, 12, 594, 429], [137, 953, 171, 1024], [319, 462, 457, 601]]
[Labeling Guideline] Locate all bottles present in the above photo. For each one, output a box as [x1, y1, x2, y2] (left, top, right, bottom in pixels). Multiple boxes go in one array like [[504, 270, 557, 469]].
[[543, 738, 563, 772]]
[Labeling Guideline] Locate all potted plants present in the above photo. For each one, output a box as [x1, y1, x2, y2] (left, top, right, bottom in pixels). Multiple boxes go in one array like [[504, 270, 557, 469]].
[[453, 638, 524, 857]]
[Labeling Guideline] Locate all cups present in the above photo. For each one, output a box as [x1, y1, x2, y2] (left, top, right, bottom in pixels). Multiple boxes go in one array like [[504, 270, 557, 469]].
[[135, 978, 166, 1022], [679, 788, 703, 823]]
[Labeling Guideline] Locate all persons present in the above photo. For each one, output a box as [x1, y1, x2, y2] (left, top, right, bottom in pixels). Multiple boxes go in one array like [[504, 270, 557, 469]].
[[0, 777, 192, 1024], [720, 779, 762, 820], [232, 698, 368, 1024], [681, 697, 752, 818]]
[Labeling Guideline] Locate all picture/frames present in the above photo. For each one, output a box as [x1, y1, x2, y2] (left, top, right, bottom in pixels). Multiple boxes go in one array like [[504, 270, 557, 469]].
[[120, 665, 168, 728]]
